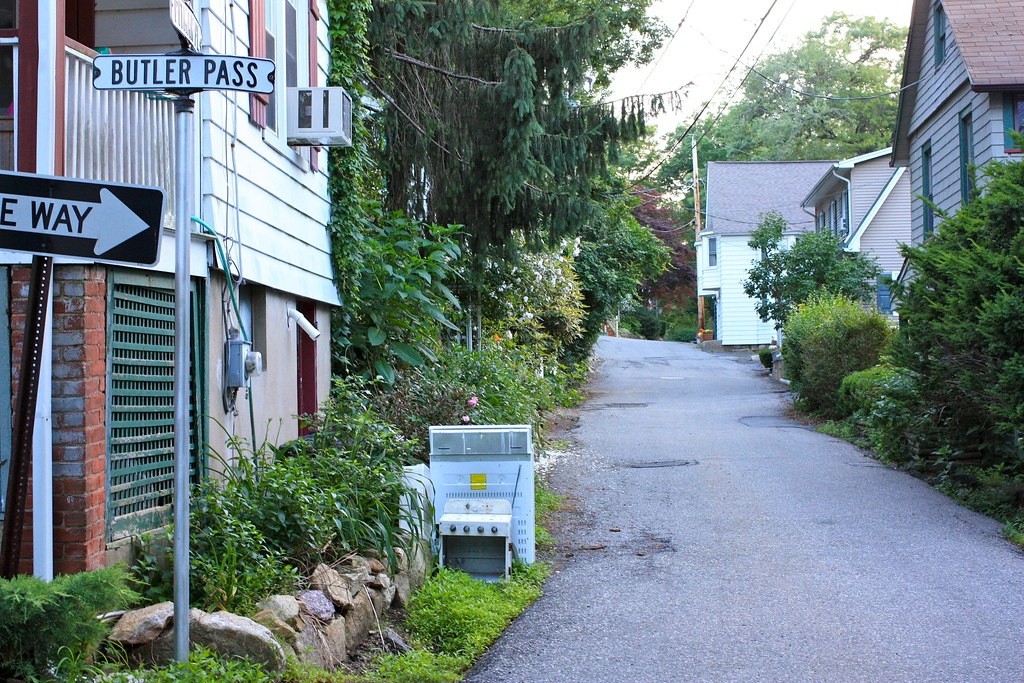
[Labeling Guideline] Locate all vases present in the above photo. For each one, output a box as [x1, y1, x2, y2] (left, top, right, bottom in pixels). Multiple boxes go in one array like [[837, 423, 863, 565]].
[[702, 329, 713, 341]]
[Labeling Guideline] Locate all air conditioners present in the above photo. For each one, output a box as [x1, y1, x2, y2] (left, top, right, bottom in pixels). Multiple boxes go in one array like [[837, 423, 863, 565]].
[[286, 86, 352, 145]]
